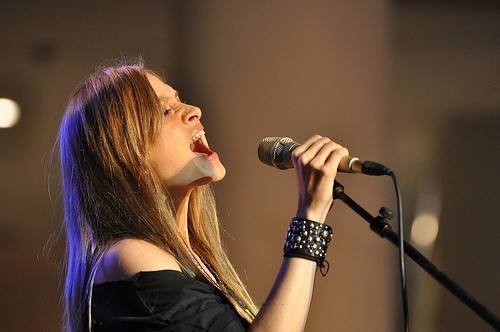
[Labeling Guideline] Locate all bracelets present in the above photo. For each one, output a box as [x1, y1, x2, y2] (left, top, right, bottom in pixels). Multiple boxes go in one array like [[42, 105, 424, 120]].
[[281, 216, 334, 278]]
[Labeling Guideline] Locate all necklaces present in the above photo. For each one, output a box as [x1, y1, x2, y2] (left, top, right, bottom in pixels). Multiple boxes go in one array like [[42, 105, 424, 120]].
[[185, 243, 258, 321]]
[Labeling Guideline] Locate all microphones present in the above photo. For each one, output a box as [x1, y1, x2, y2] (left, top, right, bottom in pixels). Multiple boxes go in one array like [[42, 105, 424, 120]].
[[258, 136, 392, 177]]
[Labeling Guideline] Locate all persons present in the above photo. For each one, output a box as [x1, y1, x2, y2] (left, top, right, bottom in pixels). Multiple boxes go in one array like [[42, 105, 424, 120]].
[[57, 63, 349, 331]]
